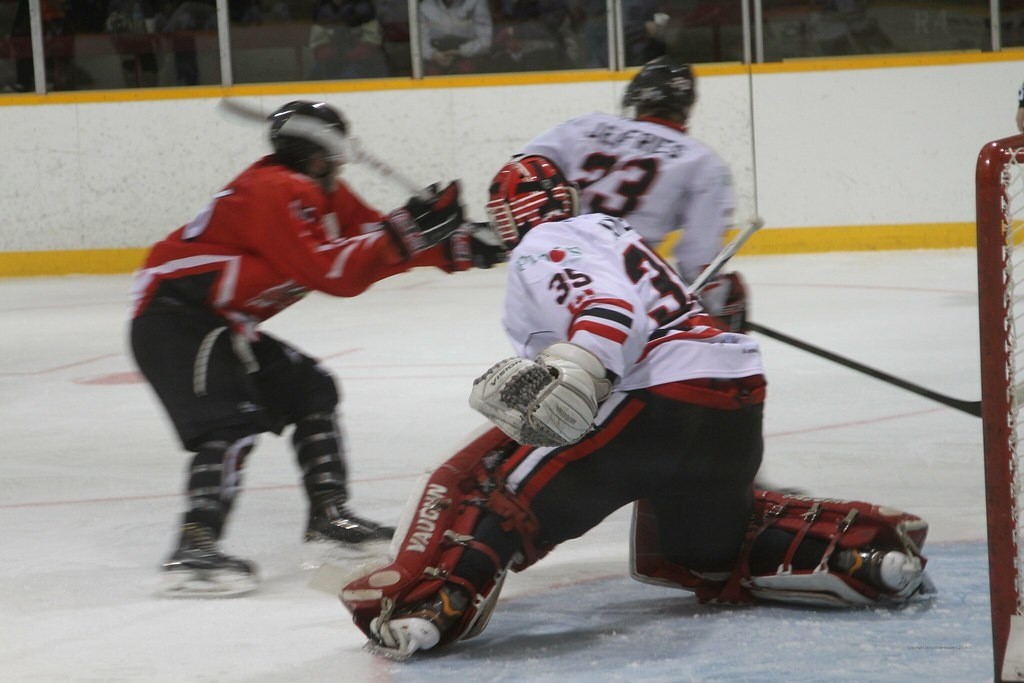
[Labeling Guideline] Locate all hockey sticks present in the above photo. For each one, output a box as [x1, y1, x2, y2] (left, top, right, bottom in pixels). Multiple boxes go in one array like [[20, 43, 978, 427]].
[[685, 216, 766, 296], [742, 320, 983, 425], [214, 93, 488, 229]]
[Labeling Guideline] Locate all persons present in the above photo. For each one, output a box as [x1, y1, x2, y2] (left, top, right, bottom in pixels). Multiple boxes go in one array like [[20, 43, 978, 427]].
[[0, 0, 683, 91], [509, 54, 735, 284], [130, 100, 507, 597], [342, 156, 928, 661]]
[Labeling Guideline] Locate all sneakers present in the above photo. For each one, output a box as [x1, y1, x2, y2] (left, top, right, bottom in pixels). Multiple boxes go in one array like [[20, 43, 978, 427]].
[[305, 501, 397, 570], [361, 587, 469, 663], [162, 521, 253, 599], [839, 549, 936, 597]]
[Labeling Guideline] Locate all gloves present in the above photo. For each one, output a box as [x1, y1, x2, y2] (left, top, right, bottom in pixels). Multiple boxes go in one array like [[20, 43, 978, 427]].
[[469, 340, 611, 448], [445, 222, 508, 271], [710, 271, 746, 334], [384, 178, 464, 258]]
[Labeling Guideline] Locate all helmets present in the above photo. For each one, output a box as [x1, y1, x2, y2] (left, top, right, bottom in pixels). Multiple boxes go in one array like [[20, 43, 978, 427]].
[[269, 99, 348, 169], [624, 56, 695, 118], [490, 150, 577, 246]]
